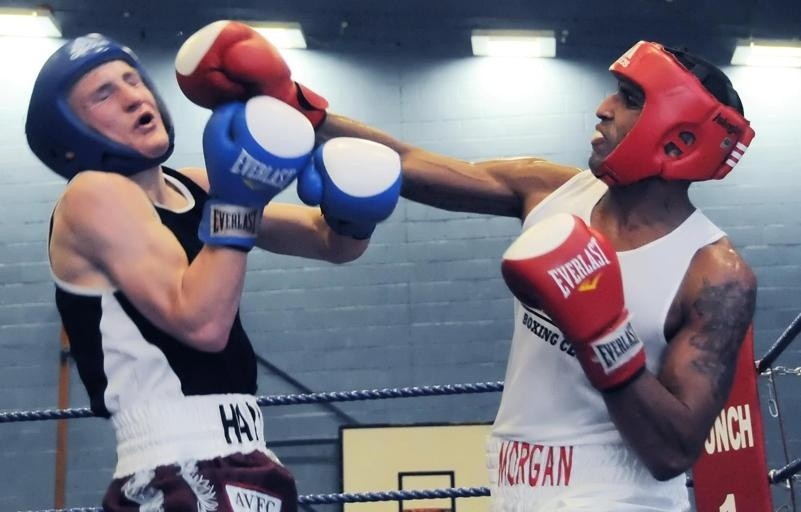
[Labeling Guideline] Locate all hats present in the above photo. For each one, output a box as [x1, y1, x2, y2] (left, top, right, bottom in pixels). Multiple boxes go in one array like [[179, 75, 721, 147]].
[[23, 29, 174, 178], [593, 37, 754, 188]]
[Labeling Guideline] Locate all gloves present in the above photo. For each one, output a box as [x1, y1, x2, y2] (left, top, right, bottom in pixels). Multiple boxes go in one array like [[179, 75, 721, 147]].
[[500, 211, 647, 391], [197, 95, 317, 253], [173, 19, 329, 130], [297, 135, 404, 242]]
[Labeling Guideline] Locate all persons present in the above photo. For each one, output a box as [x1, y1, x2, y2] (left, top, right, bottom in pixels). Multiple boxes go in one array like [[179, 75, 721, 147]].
[[173, 17, 760, 511], [21, 29, 402, 510]]
